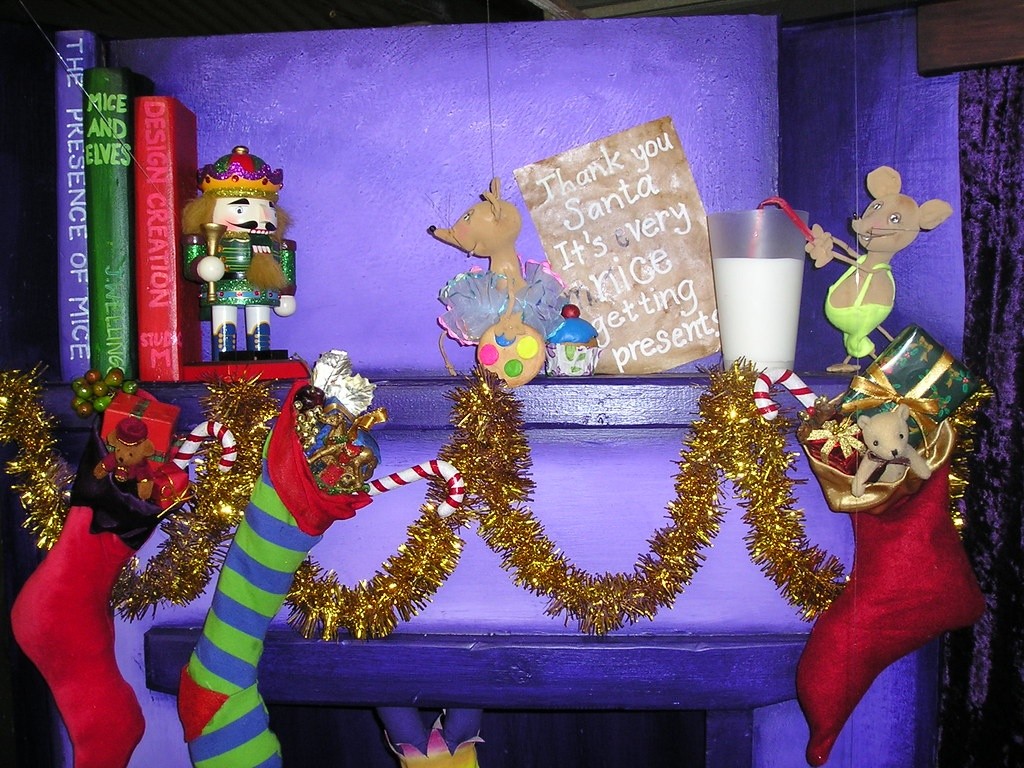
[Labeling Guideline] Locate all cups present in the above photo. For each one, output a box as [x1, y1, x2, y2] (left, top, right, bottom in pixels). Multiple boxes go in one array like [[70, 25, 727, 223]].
[[705, 210, 810, 371]]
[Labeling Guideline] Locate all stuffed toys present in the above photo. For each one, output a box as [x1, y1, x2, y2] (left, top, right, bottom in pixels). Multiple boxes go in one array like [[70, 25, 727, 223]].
[[427, 177, 566, 344], [94, 417, 155, 502], [803, 164, 953, 373], [850, 404, 932, 498]]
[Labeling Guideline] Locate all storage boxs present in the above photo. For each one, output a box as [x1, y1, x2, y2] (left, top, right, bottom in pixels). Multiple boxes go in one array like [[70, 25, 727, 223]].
[[834, 322, 975, 449], [307, 398, 381, 465], [100, 392, 183, 453]]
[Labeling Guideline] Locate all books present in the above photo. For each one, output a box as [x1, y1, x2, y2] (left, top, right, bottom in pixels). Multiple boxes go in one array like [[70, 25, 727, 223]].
[[54, 29, 201, 387]]
